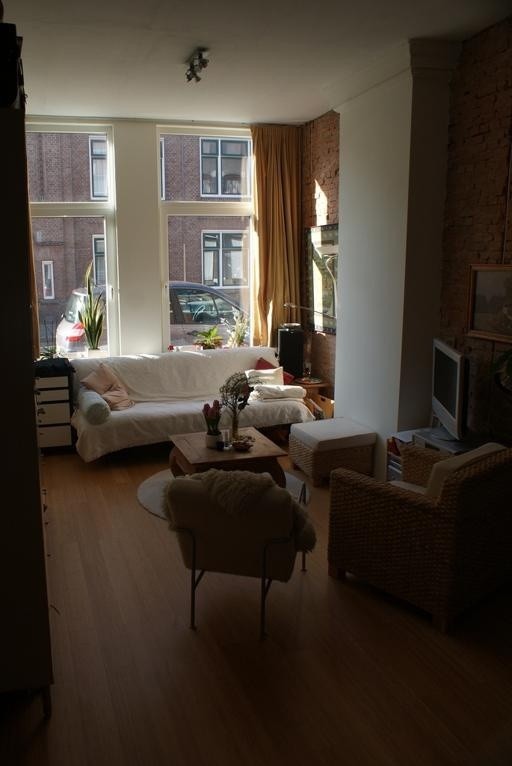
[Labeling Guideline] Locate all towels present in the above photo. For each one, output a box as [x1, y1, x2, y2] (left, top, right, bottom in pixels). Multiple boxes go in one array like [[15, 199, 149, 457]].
[[251, 385, 307, 403]]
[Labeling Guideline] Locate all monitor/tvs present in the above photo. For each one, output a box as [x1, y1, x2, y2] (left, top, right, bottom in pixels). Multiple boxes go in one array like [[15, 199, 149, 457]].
[[429, 339, 470, 441]]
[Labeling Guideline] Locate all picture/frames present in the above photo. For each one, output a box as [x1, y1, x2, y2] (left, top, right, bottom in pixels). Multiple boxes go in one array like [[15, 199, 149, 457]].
[[298, 222, 339, 338], [462, 262, 512, 346]]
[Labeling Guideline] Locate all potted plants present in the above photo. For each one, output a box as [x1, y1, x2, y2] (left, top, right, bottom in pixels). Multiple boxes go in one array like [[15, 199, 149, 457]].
[[76, 261, 105, 356], [187, 323, 225, 350], [218, 372, 253, 444]]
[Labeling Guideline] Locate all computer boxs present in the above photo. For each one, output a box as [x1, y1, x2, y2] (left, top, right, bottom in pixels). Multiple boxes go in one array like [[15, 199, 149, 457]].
[[411, 429, 480, 456]]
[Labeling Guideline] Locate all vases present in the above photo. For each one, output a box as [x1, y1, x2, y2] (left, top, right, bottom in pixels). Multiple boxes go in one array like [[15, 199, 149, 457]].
[[206, 433, 224, 448]]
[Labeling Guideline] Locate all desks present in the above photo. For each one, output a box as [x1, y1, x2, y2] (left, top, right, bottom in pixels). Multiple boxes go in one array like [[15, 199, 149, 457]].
[[295, 376, 329, 400]]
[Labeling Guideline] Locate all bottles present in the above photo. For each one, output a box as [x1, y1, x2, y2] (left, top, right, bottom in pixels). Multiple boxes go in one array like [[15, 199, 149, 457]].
[[224, 432, 230, 448], [303, 368, 310, 376]]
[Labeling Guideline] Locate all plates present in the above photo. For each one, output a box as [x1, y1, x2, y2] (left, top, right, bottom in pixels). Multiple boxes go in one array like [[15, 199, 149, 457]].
[[295, 378, 322, 384]]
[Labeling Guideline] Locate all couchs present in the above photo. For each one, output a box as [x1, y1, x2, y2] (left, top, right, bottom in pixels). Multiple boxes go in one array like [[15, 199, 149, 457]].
[[67, 346, 318, 465]]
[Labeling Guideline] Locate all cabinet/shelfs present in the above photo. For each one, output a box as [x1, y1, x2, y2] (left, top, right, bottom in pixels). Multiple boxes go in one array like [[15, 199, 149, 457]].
[[31, 362, 75, 454], [385, 428, 431, 478]]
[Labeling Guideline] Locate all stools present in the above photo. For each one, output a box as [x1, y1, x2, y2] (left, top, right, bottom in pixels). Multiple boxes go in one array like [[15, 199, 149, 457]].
[[288, 414, 379, 487]]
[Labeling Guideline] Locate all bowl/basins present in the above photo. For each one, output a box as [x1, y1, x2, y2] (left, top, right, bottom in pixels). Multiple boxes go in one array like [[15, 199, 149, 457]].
[[231, 441, 253, 451]]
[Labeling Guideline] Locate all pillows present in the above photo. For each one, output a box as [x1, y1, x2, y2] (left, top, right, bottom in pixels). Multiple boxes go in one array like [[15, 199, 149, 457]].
[[254, 357, 294, 386], [77, 386, 111, 426], [82, 362, 136, 411], [245, 365, 284, 387]]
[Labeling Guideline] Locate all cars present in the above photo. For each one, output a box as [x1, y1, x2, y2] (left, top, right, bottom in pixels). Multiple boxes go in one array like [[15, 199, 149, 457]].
[[54, 280, 249, 355]]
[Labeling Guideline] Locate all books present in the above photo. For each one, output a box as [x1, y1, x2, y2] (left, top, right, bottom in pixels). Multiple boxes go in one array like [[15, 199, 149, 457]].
[[388, 451, 402, 480]]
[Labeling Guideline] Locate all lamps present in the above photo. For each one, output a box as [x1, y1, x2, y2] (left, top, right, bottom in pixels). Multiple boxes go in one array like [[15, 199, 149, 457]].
[[181, 44, 212, 83], [283, 301, 337, 321]]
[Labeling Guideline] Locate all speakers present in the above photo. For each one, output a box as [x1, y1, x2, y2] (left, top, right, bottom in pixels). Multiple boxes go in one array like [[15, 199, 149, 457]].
[[278, 329, 303, 379]]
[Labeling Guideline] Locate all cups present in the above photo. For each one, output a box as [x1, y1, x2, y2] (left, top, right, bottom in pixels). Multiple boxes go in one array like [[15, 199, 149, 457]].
[[216, 440, 224, 450]]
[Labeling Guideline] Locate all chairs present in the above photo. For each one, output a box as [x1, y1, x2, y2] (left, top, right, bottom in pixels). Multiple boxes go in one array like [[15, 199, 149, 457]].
[[326, 443, 511, 634], [159, 468, 316, 643]]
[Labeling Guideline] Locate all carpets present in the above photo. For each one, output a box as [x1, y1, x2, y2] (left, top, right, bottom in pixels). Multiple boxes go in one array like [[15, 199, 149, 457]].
[[138, 465, 310, 522]]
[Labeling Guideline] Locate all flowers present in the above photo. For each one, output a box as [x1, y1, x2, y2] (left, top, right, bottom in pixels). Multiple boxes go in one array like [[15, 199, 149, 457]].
[[202, 400, 223, 435]]
